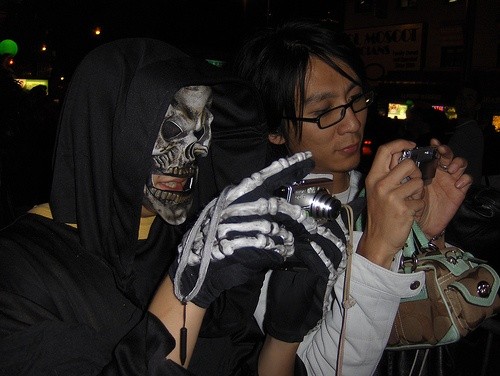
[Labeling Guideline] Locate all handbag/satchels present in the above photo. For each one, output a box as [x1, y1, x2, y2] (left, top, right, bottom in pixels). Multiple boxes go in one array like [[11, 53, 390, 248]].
[[351, 186, 500, 347]]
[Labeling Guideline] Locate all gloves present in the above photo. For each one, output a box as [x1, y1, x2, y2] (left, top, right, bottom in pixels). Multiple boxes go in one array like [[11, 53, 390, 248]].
[[261, 200, 352, 343], [168, 149, 320, 310]]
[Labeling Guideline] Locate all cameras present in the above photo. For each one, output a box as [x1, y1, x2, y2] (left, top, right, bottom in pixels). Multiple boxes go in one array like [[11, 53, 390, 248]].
[[399, 147, 440, 184], [274, 178, 341, 221]]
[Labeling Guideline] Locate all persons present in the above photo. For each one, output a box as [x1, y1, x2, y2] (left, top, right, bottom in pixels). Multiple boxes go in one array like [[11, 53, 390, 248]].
[[1, 39, 350, 375], [222, 36, 473, 376]]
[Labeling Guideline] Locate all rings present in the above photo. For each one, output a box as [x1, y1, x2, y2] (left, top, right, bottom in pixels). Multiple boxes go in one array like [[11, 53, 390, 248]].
[[438, 163, 448, 170]]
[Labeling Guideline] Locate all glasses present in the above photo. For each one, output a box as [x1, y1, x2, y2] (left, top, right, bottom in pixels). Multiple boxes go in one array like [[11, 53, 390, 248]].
[[278, 90, 374, 129]]
